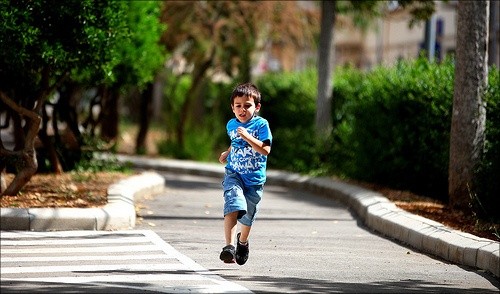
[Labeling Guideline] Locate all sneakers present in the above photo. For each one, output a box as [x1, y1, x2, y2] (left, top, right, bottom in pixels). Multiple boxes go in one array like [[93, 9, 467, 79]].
[[219, 245, 236, 263], [236, 232, 249, 265]]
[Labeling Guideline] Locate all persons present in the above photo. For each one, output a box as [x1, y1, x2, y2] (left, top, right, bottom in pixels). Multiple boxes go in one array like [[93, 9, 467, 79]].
[[218, 81, 273, 265]]
